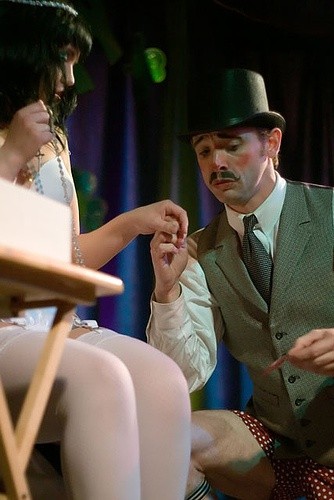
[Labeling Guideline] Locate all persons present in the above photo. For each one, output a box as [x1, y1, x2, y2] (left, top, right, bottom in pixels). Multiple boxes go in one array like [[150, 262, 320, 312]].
[[0, 1, 193, 499], [145, 69, 334, 499]]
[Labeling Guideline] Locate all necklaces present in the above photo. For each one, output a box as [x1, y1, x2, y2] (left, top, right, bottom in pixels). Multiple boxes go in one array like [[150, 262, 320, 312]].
[[4, 120, 88, 268]]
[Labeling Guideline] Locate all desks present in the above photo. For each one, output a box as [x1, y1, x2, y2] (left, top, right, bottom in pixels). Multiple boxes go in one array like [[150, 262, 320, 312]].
[[0, 247, 126, 500]]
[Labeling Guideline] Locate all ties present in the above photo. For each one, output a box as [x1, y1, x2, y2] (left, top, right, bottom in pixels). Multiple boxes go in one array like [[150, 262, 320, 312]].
[[241, 214, 273, 306]]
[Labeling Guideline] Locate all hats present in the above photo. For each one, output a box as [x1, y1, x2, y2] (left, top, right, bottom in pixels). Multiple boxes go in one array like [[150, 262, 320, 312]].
[[176, 69, 286, 141]]
